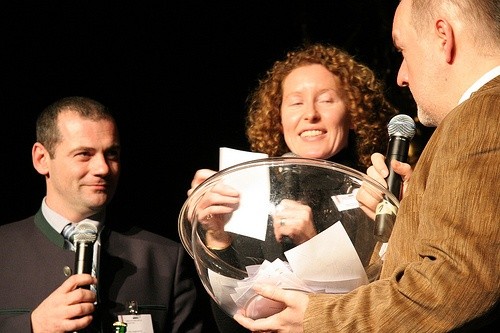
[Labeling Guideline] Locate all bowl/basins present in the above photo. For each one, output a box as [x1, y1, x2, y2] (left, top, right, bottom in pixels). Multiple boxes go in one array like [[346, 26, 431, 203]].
[[178, 158, 399, 320]]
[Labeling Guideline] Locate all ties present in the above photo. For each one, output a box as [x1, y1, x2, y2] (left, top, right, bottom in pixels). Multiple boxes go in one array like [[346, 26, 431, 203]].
[[63, 225, 97, 292]]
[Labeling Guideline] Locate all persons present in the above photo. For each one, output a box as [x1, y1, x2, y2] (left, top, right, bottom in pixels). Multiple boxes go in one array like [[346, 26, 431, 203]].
[[187, 43, 400, 333], [233, 0, 500, 333], [0, 96, 218, 333]]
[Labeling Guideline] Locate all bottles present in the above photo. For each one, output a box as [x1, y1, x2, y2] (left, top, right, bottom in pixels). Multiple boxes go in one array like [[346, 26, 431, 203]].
[[113, 322, 127, 333]]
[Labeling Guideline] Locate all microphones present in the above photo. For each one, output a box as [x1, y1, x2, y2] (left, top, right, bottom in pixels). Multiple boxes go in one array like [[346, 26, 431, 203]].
[[68, 221, 99, 333], [374, 113, 415, 242], [279, 152, 301, 244]]
